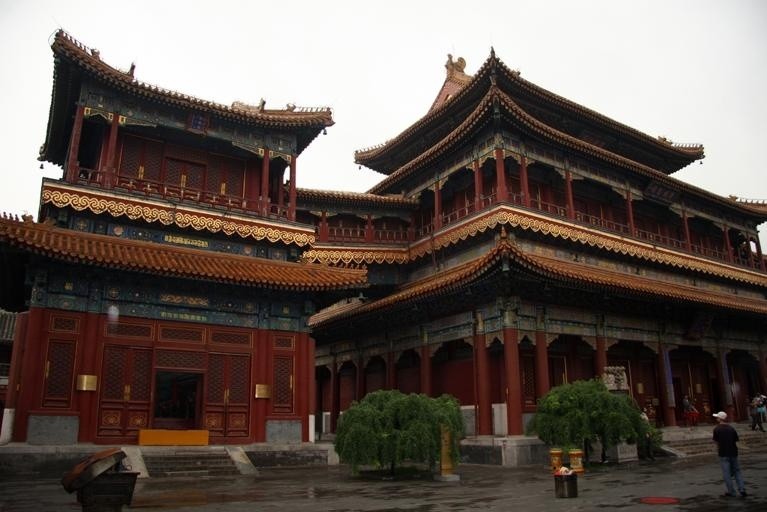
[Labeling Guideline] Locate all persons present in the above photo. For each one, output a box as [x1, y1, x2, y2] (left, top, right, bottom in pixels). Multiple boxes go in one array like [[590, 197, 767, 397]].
[[712, 411, 747, 497], [748, 392, 767, 433], [640, 407, 656, 461], [682, 393, 690, 413]]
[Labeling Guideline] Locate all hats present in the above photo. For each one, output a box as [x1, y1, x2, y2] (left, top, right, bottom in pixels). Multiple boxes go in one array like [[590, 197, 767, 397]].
[[754, 395, 766, 401], [712, 411, 727, 420]]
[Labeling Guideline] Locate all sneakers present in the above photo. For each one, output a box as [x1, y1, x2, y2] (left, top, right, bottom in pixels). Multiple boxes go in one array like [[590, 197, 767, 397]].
[[752, 428, 767, 432], [725, 492, 747, 497]]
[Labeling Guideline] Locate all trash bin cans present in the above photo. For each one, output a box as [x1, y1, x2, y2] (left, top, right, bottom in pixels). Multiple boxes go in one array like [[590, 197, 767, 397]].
[[555, 475, 578, 498]]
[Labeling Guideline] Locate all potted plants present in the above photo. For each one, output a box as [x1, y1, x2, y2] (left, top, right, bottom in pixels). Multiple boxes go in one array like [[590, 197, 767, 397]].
[[547, 446, 584, 474]]
